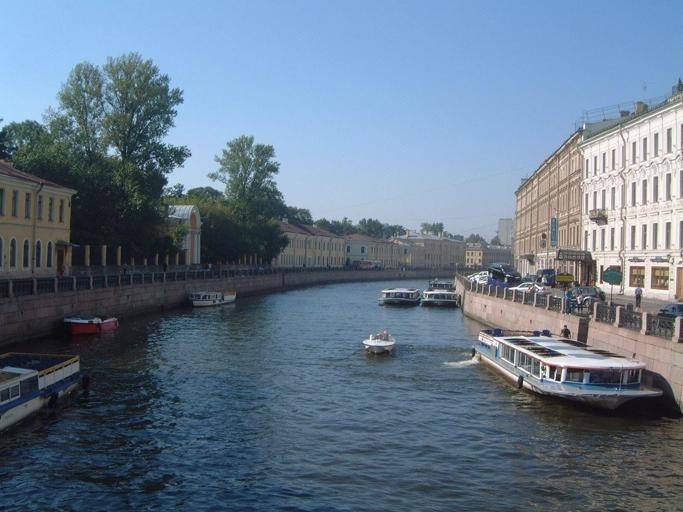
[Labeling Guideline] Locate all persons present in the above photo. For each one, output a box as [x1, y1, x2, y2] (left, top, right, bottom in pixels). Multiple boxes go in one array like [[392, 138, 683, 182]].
[[560, 325, 571, 339], [635, 284, 642, 307]]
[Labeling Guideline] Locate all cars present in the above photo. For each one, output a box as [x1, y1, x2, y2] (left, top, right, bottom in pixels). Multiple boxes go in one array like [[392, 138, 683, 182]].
[[506, 282, 552, 298], [658, 303, 683, 324], [486, 263, 522, 285], [466, 270, 490, 287]]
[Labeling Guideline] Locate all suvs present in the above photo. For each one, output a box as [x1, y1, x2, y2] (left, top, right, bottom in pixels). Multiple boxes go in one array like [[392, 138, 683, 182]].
[[554, 286, 606, 308]]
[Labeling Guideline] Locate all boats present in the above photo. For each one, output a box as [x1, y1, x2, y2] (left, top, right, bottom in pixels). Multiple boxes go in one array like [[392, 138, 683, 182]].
[[63, 314, 119, 337], [0, 350, 90, 439], [470, 329, 663, 412], [422, 279, 461, 309], [377, 287, 421, 307], [188, 290, 237, 307], [362, 329, 396, 354]]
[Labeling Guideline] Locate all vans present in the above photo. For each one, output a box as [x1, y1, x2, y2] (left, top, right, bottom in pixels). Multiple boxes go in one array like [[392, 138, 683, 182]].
[[536, 269, 555, 288]]
